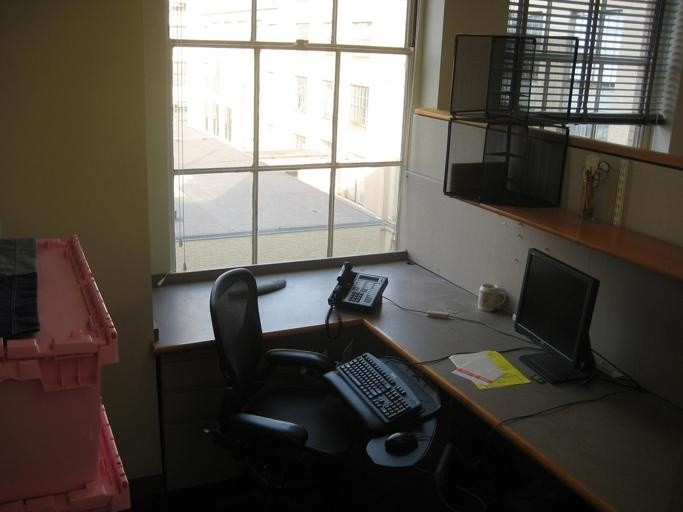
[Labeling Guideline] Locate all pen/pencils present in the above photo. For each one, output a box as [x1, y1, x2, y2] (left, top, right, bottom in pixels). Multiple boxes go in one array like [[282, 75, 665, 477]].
[[580, 165, 594, 219]]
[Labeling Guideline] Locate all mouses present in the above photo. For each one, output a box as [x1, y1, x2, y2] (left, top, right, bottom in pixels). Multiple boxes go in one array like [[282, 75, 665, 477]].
[[385, 431, 419, 457]]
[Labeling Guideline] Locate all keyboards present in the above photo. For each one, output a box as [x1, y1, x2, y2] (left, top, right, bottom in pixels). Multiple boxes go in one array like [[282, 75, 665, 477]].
[[337, 353, 421, 424]]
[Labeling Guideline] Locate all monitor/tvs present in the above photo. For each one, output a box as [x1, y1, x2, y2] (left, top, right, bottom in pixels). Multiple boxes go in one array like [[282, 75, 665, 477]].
[[515, 248, 600, 383]]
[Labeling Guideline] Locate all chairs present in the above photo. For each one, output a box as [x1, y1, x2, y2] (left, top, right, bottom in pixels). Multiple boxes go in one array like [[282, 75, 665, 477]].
[[210, 268, 439, 497]]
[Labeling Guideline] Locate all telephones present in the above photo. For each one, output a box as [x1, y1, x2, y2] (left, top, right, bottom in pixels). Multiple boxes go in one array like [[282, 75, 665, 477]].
[[328, 262, 388, 314]]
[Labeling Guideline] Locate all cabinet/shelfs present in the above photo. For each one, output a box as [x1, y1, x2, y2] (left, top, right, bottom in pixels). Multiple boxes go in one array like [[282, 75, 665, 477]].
[[402, 112, 683, 405]]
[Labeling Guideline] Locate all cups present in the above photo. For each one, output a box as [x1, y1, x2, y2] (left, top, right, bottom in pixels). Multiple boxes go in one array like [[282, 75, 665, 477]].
[[477, 283, 507, 313]]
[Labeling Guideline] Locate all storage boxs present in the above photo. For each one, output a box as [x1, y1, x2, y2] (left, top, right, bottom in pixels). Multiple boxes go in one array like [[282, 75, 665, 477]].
[[1, 235, 119, 505], [0, 404, 131, 512]]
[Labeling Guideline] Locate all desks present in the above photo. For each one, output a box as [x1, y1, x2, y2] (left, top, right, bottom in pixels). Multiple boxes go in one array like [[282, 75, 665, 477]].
[[153, 250, 683, 512]]
